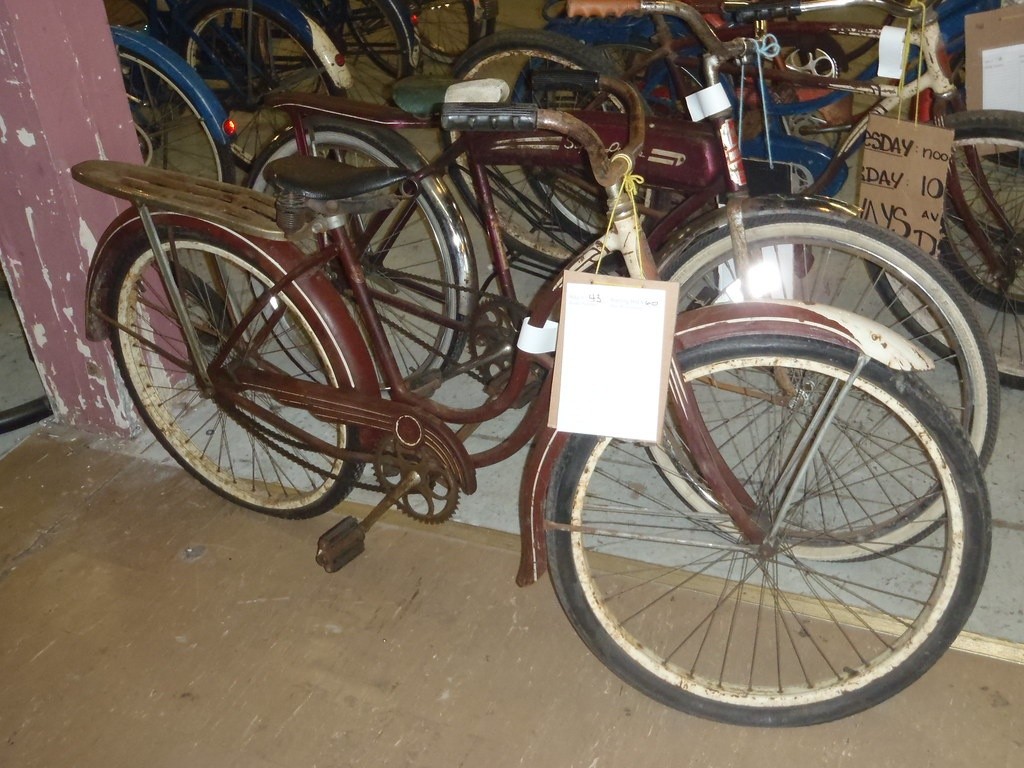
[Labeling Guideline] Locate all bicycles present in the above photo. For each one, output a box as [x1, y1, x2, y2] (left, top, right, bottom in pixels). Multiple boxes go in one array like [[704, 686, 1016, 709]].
[[72, 0, 1024, 733]]
